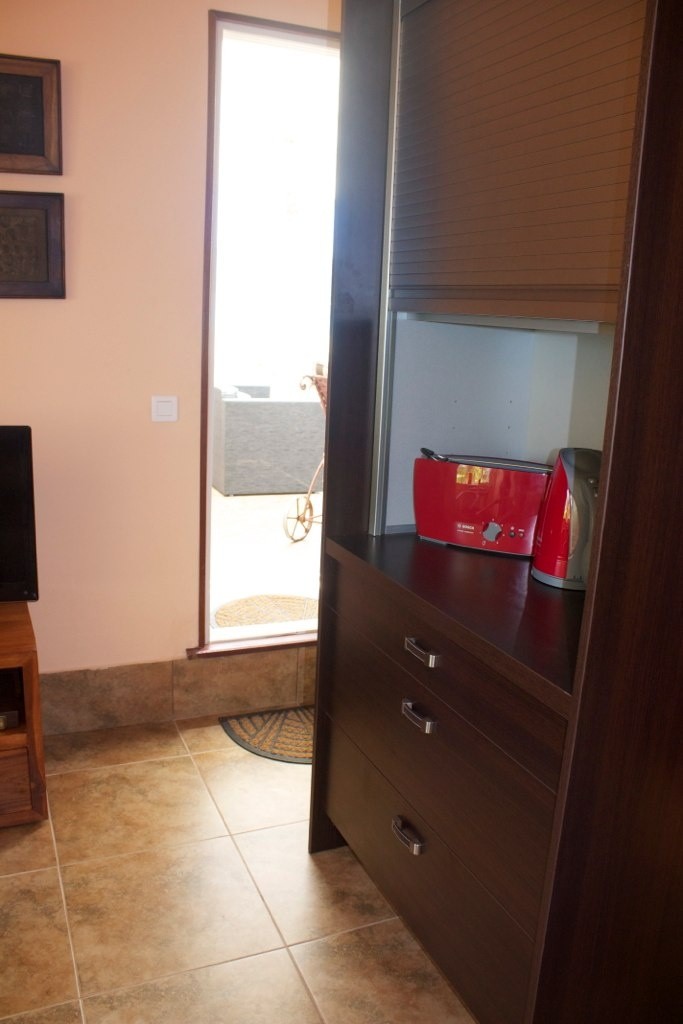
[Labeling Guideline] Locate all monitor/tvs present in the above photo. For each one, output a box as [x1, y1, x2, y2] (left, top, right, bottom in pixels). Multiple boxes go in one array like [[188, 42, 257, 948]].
[[0, 425, 38, 605]]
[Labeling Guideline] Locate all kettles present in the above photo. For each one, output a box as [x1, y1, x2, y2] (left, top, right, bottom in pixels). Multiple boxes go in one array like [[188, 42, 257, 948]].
[[532, 447, 601, 591]]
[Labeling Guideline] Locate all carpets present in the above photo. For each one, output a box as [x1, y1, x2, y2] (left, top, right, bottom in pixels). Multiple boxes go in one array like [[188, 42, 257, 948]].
[[219, 704, 314, 765]]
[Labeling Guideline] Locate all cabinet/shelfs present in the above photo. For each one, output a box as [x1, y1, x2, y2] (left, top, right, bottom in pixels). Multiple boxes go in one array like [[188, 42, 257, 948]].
[[0, 600, 50, 826], [310, 0, 683, 1024]]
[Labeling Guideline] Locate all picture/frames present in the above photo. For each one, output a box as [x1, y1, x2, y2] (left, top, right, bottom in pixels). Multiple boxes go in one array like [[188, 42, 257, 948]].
[[0, 54, 63, 175], [0, 190, 66, 299]]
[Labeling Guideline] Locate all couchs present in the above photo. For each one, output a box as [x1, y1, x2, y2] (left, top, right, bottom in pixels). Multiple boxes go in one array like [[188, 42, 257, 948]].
[[208, 383, 324, 495]]
